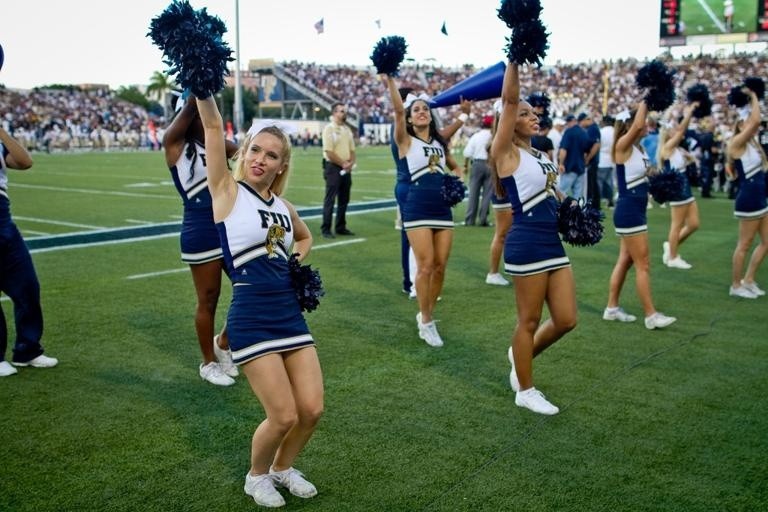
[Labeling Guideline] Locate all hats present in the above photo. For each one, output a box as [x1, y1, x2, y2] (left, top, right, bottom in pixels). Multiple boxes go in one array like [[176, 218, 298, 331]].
[[481, 116, 494, 128]]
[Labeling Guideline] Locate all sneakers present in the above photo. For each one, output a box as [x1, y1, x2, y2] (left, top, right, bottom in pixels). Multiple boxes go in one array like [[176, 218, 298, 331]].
[[199, 361, 236, 387], [243, 472, 286, 508], [11, 353, 58, 369], [605, 203, 616, 209], [647, 203, 655, 208], [515, 387, 560, 416], [1, 362, 18, 378], [213, 335, 241, 378], [645, 312, 678, 330], [416, 313, 444, 347], [667, 255, 693, 269], [742, 278, 767, 297], [485, 273, 510, 286], [728, 285, 757, 300], [662, 240, 670, 263], [660, 202, 666, 209], [270, 464, 317, 499], [419, 325, 426, 341], [602, 307, 637, 323], [507, 345, 524, 392]]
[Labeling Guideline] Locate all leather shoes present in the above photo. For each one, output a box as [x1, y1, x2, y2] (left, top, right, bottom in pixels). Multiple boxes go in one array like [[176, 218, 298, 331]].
[[480, 221, 492, 227], [321, 231, 336, 240], [335, 229, 356, 235], [460, 221, 472, 227]]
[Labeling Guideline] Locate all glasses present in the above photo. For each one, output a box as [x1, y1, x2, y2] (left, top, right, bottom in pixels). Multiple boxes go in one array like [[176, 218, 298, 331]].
[[336, 109, 345, 114]]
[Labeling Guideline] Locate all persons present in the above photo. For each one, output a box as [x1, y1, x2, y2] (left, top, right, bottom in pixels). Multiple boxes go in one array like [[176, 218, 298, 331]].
[[196, 85, 324, 508], [390, 87, 473, 298], [472, 98, 493, 115], [386, 74, 462, 347], [583, 114, 601, 204], [532, 117, 554, 163], [399, 64, 425, 87], [561, 115, 576, 136], [162, 89, 240, 386], [655, 49, 709, 97], [710, 53, 726, 144], [727, 86, 768, 301], [603, 98, 677, 330], [656, 102, 700, 270], [356, 103, 385, 124], [726, 51, 767, 85], [425, 74, 455, 96], [485, 46, 578, 415], [519, 56, 648, 112], [759, 88, 768, 147], [485, 193, 513, 286], [597, 115, 615, 208], [437, 104, 461, 127], [320, 103, 356, 238], [0, 43, 58, 379], [1, 86, 168, 154], [558, 113, 591, 204], [283, 59, 318, 84], [463, 116, 496, 226], [320, 65, 388, 103]]
